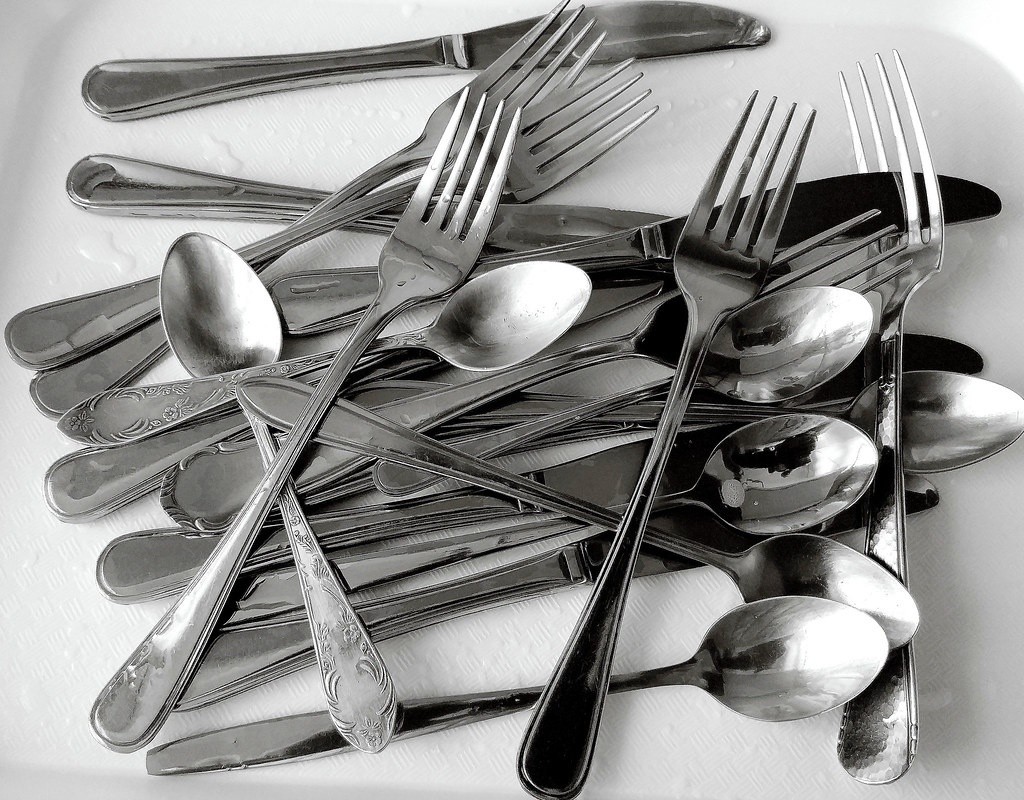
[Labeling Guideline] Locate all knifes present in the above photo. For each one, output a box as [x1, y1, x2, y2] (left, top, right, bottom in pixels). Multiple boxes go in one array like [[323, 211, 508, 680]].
[[66, 0, 1001, 718]]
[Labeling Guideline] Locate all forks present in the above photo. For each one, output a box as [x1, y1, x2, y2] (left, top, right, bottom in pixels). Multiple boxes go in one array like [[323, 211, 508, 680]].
[[18, 0, 946, 797]]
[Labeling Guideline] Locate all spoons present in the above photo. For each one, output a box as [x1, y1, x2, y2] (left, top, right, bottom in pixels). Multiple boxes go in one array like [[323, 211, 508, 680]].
[[42, 230, 1023, 787]]
